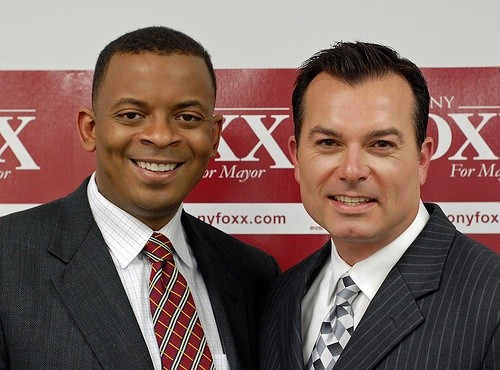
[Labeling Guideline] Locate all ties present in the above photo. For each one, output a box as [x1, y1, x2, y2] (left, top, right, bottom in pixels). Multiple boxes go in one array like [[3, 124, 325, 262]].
[[303, 270, 362, 370], [140, 232, 216, 370]]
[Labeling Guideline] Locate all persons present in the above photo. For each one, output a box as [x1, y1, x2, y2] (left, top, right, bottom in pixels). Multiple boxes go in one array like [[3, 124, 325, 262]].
[[0, 27, 281, 370], [256, 42, 500, 370]]
